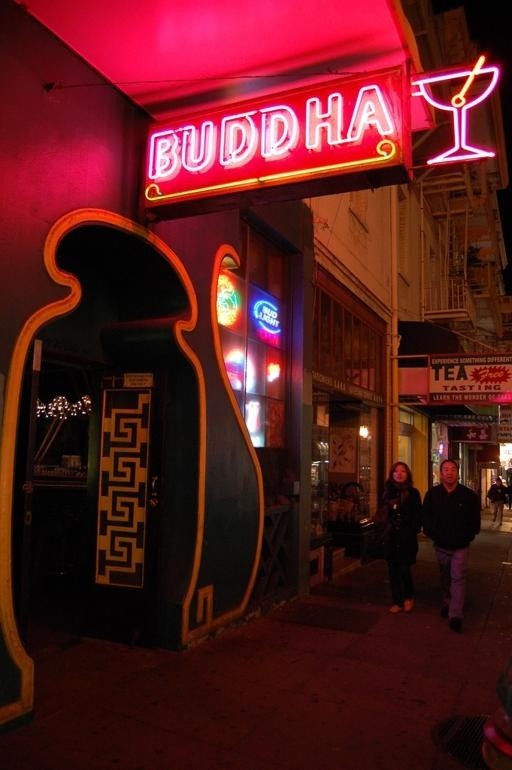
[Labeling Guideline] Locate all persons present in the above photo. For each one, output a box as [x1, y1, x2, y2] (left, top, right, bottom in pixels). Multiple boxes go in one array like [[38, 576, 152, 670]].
[[371, 462, 423, 615], [485, 477, 507, 526], [422, 458, 483, 634]]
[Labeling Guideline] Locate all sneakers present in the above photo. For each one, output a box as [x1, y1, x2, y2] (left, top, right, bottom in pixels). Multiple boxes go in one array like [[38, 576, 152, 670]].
[[446, 617, 465, 634], [440, 604, 451, 620], [388, 599, 416, 613]]
